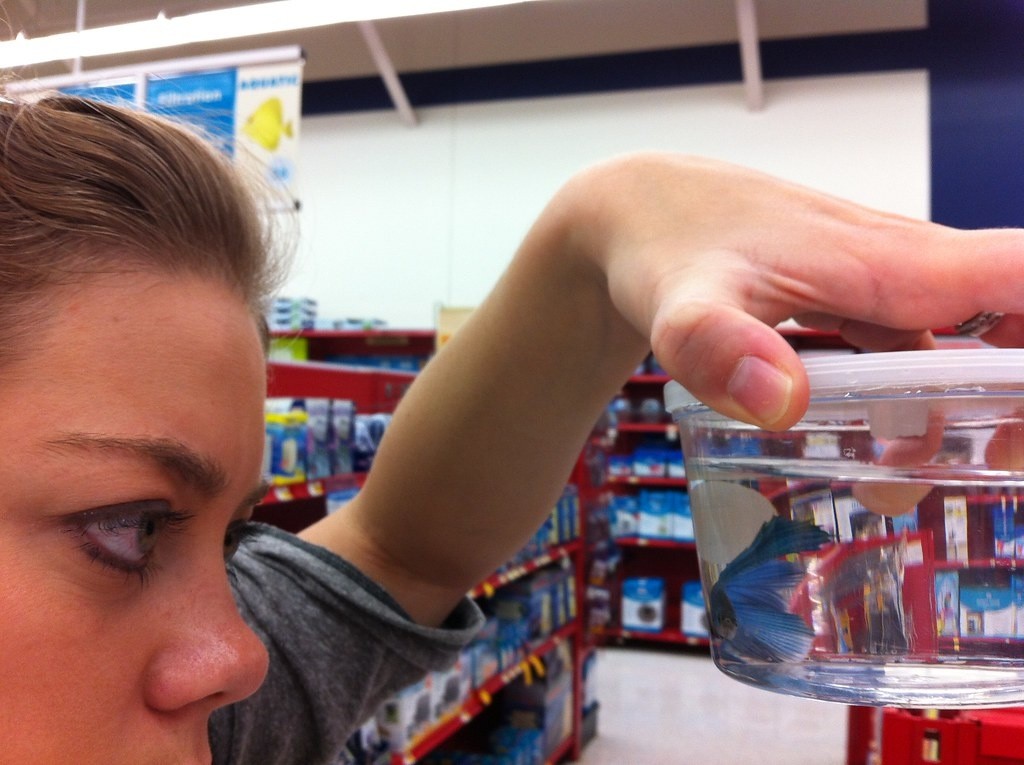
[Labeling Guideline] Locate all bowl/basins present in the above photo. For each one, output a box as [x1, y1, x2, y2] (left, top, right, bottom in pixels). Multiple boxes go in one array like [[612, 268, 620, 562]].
[[651, 342, 1023, 713]]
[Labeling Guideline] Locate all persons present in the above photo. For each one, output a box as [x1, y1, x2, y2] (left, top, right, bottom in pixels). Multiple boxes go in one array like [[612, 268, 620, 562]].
[[0, 94, 1024, 765]]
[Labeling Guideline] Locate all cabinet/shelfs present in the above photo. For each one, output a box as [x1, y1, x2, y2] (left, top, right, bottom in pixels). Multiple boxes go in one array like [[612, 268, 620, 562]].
[[269, 329, 1024, 656], [257, 359, 615, 765]]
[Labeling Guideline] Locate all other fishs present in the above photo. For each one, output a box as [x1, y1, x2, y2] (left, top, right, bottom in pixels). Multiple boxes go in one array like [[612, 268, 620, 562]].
[[707, 517, 832, 666]]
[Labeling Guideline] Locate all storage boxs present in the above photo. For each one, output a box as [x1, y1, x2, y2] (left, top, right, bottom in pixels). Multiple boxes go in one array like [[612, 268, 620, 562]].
[[1010, 574, 1024, 638], [935, 571, 960, 636], [960, 585, 1013, 637], [892, 504, 917, 534], [263, 354, 708, 764], [943, 496, 969, 562], [993, 504, 1016, 558], [1014, 523, 1024, 560], [834, 495, 887, 543]]
